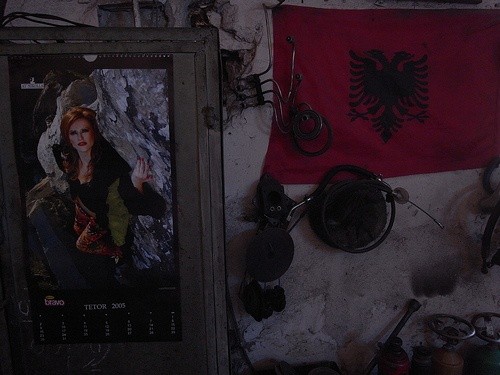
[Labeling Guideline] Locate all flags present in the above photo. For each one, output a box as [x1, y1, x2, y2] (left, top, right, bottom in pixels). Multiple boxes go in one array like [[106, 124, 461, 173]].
[[263, 5, 500, 185]]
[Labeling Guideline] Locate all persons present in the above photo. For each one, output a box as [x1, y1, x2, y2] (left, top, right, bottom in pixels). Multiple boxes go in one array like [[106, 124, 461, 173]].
[[52, 107, 153, 258]]
